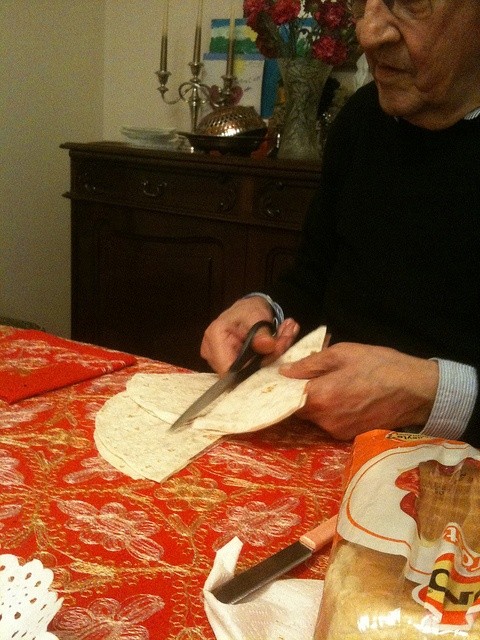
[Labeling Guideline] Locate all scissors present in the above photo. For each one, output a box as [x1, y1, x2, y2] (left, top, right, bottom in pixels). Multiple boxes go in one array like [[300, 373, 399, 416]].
[[167, 322, 280, 435]]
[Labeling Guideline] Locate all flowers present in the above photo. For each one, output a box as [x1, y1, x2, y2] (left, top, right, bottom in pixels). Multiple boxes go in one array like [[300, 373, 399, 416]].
[[243, 0, 359, 59]]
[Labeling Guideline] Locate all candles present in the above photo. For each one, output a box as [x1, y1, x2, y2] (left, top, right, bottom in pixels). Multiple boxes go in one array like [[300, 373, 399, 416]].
[[190, 0, 206, 85], [223, 2, 237, 94], [159, 0, 170, 97]]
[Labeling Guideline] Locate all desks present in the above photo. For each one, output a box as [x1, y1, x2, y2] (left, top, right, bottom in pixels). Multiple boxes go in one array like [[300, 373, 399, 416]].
[[1, 324, 355, 635]]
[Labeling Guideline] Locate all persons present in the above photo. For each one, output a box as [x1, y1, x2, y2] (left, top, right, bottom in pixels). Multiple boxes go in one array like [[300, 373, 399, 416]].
[[199, 2, 480, 443]]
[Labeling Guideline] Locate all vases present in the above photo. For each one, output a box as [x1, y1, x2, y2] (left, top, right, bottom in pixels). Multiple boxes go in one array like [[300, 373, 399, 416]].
[[275, 59, 333, 167]]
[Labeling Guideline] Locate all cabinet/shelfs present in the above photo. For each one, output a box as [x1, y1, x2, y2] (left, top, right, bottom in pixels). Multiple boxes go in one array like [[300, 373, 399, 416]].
[[243, 163, 314, 294], [70, 151, 241, 375]]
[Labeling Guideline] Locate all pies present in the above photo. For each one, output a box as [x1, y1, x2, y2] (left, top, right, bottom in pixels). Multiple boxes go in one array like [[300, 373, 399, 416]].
[[92, 325, 333, 483]]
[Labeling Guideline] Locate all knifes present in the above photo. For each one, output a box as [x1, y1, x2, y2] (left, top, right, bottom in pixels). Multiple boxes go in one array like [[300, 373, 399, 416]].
[[214, 514, 339, 606]]
